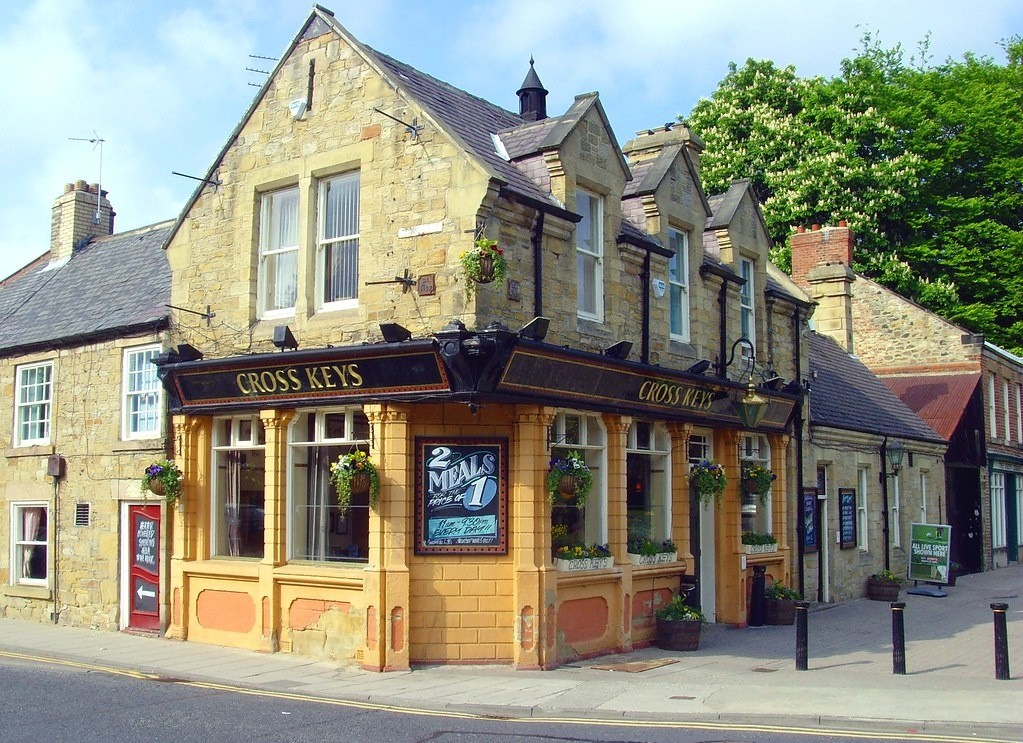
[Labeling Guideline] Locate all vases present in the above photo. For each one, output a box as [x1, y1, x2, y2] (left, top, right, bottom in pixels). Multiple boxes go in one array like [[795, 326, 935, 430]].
[[689, 475, 720, 492], [148, 477, 171, 494], [558, 474, 583, 498], [762, 595, 796, 625], [928, 569, 958, 587], [474, 254, 496, 283], [658, 617, 702, 652], [345, 466, 371, 491], [554, 535, 568, 547], [744, 477, 770, 494], [555, 554, 615, 571], [627, 550, 677, 564], [870, 581, 901, 601]]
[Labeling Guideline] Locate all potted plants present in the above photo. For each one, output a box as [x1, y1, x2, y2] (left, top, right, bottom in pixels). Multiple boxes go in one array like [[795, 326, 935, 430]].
[[742, 531, 778, 555]]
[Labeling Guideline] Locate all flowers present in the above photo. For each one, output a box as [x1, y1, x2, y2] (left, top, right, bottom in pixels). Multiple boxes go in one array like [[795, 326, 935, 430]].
[[762, 579, 799, 599], [547, 451, 593, 509], [654, 594, 705, 620], [551, 525, 568, 541], [557, 543, 612, 560], [627, 536, 678, 556], [141, 458, 182, 501], [949, 558, 961, 571], [740, 463, 775, 480], [328, 450, 380, 515], [684, 459, 727, 482], [873, 569, 903, 583], [460, 236, 506, 294]]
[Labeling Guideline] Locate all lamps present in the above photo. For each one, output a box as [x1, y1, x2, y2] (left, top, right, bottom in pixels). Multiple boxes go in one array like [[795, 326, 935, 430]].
[[604, 340, 633, 359], [736, 358, 769, 428], [878, 439, 905, 481], [521, 316, 551, 341], [379, 321, 413, 344], [688, 360, 711, 374], [766, 375, 785, 391], [273, 325, 297, 352], [177, 343, 203, 363]]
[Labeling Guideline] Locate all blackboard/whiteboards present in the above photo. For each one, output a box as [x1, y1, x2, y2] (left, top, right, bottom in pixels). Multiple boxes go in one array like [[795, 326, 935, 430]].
[[803, 487, 822, 553], [838, 487, 857, 549], [415, 437, 508, 555]]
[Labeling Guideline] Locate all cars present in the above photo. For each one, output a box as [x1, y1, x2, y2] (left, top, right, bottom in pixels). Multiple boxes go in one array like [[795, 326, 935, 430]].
[[237, 506, 265, 534]]
